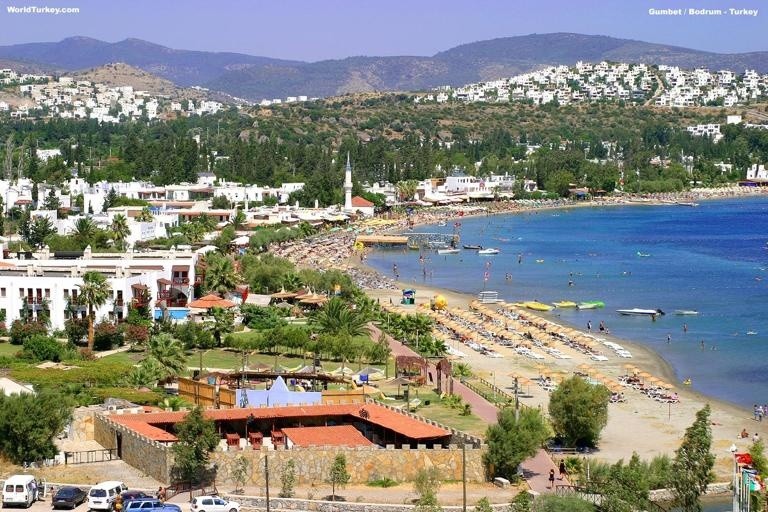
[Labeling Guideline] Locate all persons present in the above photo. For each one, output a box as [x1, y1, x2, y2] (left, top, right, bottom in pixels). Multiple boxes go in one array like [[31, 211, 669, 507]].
[[359, 205, 632, 288], [740, 403, 768, 446], [158, 493, 166, 502], [547, 467, 556, 489], [558, 458, 565, 480], [49, 486, 57, 505], [115, 490, 122, 512], [158, 485, 166, 496]]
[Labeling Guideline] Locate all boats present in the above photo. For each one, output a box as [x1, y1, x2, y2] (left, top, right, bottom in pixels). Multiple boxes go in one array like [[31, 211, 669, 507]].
[[671, 309, 699, 317], [677, 202, 699, 207], [517, 300, 552, 312], [552, 300, 576, 308], [613, 306, 659, 317], [576, 303, 601, 311], [407, 240, 500, 257]]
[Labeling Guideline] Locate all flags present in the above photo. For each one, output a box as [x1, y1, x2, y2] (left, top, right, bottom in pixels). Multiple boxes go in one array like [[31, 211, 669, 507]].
[[733, 452, 766, 493]]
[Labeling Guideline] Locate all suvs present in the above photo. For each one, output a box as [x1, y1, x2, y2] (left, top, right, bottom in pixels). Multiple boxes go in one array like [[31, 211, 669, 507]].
[[0, 473, 244, 512]]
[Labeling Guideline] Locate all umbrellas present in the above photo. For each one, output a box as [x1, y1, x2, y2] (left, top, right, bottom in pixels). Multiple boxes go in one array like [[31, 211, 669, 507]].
[[379, 296, 674, 393]]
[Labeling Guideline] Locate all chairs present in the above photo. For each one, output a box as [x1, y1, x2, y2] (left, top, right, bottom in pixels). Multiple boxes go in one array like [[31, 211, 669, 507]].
[[425, 301, 678, 410]]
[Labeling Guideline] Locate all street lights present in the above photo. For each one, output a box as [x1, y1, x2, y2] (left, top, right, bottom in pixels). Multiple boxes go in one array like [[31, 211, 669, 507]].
[[488, 367, 497, 401]]
